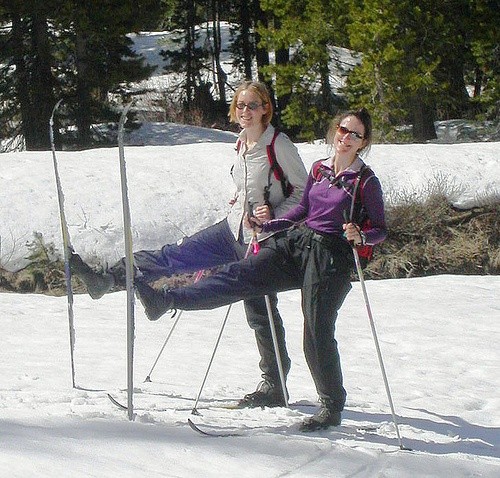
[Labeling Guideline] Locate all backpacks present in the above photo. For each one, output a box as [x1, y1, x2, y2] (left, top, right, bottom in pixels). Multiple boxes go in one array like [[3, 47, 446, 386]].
[[302, 159, 373, 273]]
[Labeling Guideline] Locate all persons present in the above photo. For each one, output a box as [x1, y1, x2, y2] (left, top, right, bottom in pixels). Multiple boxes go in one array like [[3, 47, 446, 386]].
[[68, 81, 308, 410], [131, 107, 388, 433]]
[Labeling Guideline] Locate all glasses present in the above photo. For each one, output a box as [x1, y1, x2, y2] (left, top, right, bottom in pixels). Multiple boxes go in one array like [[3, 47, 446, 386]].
[[235, 102, 266, 111], [336, 124, 366, 143]]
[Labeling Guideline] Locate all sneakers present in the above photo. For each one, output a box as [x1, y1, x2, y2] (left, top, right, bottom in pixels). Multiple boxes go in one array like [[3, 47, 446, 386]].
[[69, 254, 121, 300], [303, 399, 342, 427], [132, 276, 177, 322], [244, 374, 289, 407]]
